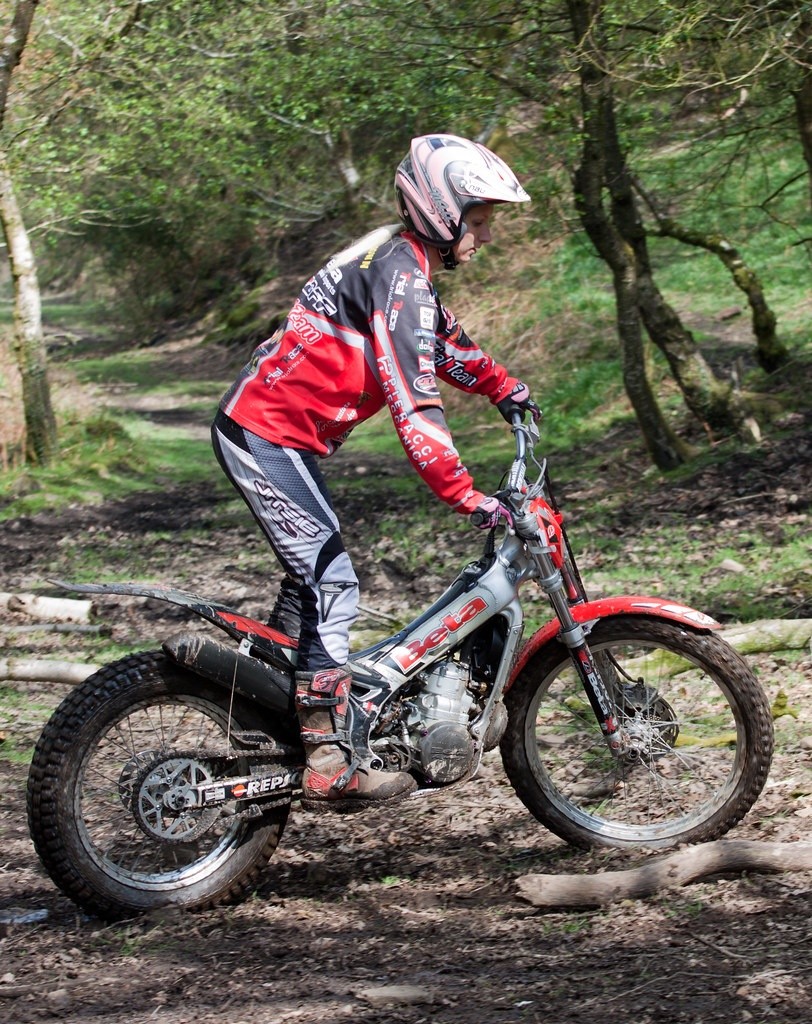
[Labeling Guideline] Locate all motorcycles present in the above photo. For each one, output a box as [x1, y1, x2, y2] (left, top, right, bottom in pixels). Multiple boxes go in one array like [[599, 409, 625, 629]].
[[26, 404, 775, 922]]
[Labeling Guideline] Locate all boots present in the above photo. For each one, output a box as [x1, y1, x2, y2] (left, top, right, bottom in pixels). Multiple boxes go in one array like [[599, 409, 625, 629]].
[[294, 664, 418, 813]]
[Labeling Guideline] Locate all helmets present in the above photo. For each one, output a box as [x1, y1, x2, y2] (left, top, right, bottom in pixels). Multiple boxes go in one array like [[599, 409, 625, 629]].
[[395, 133, 531, 248]]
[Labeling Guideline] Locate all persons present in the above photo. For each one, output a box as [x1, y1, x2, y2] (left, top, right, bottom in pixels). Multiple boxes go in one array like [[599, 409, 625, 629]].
[[210, 133, 542, 813]]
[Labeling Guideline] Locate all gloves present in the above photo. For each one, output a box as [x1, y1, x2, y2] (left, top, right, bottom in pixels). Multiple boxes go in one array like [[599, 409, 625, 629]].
[[475, 496, 517, 530], [509, 379, 542, 422]]
